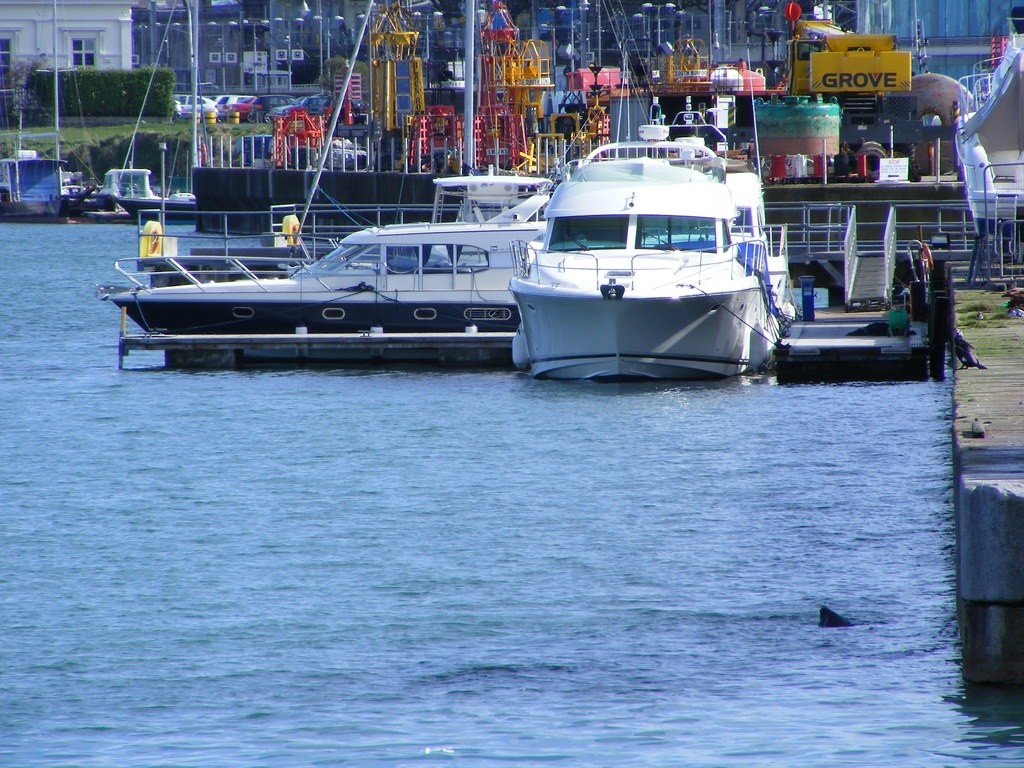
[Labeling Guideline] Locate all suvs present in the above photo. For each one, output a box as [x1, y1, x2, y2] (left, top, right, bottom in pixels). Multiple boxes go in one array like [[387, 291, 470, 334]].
[[267, 94, 332, 121]]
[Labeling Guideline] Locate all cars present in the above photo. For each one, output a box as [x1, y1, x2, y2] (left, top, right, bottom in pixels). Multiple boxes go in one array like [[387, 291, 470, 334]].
[[207, 95, 254, 117], [252, 94, 295, 122], [229, 97, 271, 123], [173, 95, 219, 120]]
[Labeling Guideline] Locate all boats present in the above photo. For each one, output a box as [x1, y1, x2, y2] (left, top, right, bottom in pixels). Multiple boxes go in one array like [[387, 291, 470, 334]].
[[772, 239, 953, 383], [0, 0, 95, 217], [506, 95, 801, 382], [62, 171, 131, 218], [95, 163, 555, 334], [954, 34, 1024, 242], [98, 168, 197, 218]]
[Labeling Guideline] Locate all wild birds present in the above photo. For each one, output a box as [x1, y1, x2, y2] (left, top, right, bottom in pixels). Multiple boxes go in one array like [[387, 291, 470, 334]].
[[955, 335, 987, 370]]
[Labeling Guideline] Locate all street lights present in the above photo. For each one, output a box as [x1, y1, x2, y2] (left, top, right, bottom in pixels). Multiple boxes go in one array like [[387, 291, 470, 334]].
[[356, 13, 385, 121], [243, 20, 269, 90], [313, 14, 344, 85], [274, 17, 304, 93], [207, 20, 238, 91], [759, 5, 789, 42], [543, 24, 556, 71], [556, 5, 589, 73], [634, 13, 652, 62], [678, 10, 694, 39], [643, 3, 676, 48], [155, 21, 181, 68], [410, 11, 443, 61]]
